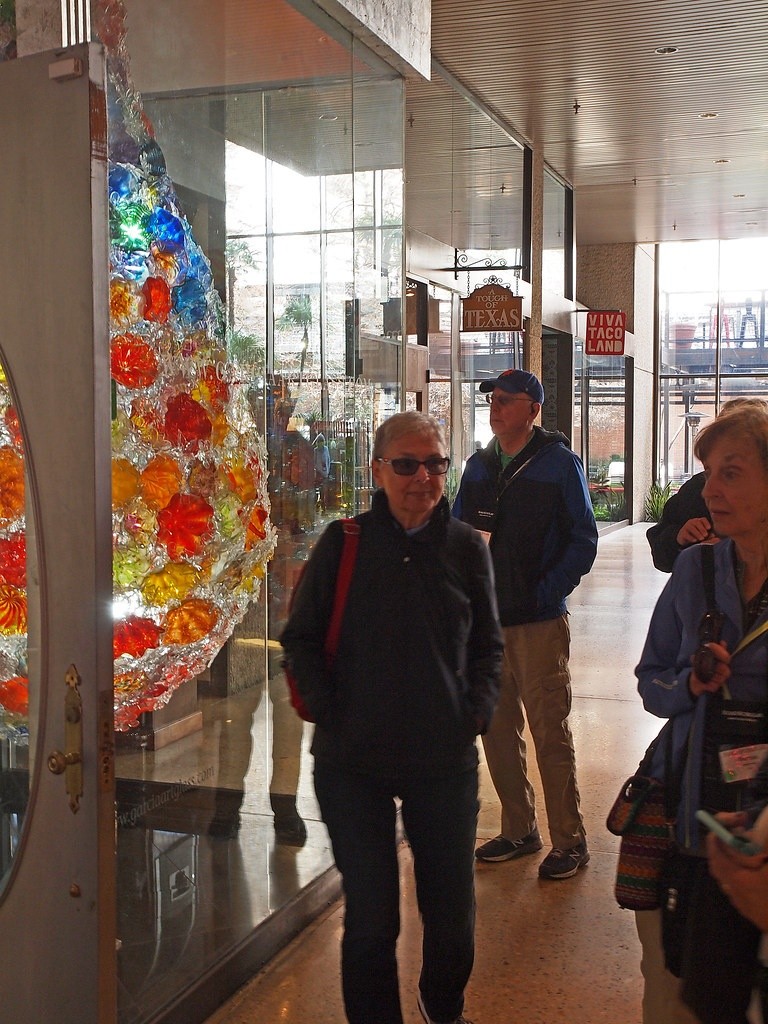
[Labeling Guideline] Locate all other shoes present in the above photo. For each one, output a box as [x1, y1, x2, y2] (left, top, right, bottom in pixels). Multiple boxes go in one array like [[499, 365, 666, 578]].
[[418, 991, 475, 1024]]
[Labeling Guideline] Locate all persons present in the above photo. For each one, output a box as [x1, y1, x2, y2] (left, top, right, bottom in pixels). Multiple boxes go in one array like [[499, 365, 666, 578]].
[[275, 411, 508, 1024], [646, 397, 768, 573], [450, 369, 598, 882], [635, 404, 768, 1024]]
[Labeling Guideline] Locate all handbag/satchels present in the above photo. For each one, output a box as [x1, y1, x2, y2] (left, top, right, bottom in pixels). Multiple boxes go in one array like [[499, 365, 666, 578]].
[[283, 518, 359, 723], [604, 774, 678, 912]]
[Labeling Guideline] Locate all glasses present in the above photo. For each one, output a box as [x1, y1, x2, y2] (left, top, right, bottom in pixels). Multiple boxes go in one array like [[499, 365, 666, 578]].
[[694, 609, 727, 683], [486, 394, 533, 405], [376, 458, 451, 476]]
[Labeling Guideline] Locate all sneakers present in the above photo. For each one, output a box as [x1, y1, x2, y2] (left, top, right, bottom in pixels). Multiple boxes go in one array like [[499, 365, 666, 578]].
[[474, 826, 543, 863], [207, 810, 240, 838], [270, 797, 307, 842], [539, 839, 590, 879]]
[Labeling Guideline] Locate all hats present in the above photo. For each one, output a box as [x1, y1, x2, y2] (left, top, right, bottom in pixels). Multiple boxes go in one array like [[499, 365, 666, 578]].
[[479, 369, 544, 406]]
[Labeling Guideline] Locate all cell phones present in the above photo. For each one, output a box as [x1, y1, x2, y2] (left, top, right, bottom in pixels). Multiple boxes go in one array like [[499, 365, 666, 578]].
[[695, 810, 759, 856]]
